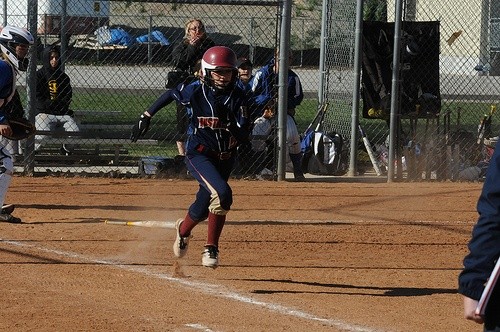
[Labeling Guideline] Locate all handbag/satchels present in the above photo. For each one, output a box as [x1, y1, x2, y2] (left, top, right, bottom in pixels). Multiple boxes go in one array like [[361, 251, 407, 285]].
[[165, 71, 189, 90]]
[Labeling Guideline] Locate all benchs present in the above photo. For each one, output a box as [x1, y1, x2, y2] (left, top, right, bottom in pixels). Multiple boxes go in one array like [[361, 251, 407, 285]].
[[13, 109, 159, 167]]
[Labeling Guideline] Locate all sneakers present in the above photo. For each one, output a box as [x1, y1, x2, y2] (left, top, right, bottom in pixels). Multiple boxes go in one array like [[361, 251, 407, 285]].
[[173, 218, 193, 259], [202, 243, 219, 269]]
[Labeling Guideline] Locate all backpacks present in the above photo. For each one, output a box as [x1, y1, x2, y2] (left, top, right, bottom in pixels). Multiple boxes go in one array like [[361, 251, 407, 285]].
[[297, 129, 347, 175]]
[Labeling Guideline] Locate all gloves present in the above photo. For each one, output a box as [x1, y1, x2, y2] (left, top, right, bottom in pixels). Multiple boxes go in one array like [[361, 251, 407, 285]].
[[130, 112, 152, 144]]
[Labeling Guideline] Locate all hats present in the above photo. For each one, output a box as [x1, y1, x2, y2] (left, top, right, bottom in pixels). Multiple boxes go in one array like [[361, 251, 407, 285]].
[[237, 57, 252, 67]]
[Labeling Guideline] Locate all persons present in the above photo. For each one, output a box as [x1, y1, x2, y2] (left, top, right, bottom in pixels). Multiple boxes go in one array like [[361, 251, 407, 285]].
[[458, 138, 500, 332], [34, 45, 80, 156], [236, 47, 306, 181], [173, 20, 216, 156], [130, 45, 248, 268], [0, 24, 35, 222]]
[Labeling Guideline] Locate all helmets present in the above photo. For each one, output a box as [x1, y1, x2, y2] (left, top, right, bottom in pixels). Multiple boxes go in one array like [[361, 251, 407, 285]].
[[200, 45, 239, 83], [0, 25, 34, 72]]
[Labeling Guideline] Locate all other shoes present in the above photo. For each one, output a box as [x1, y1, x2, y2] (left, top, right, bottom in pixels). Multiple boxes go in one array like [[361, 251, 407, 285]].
[[0, 203, 22, 223], [296, 175, 304, 181]]
